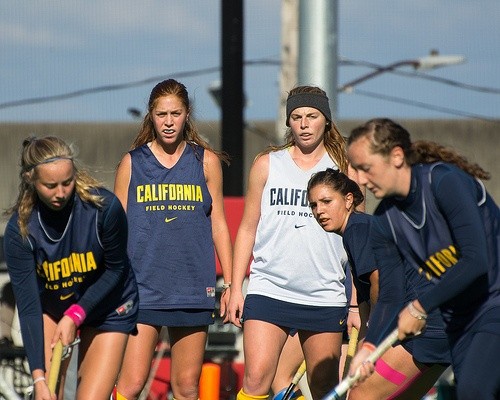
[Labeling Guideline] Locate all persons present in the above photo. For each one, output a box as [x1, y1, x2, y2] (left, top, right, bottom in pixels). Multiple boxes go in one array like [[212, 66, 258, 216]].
[[347, 117, 500, 400], [110, 79, 234, 400], [229, 86, 367, 400], [307, 168, 453, 399], [5, 135, 140, 400]]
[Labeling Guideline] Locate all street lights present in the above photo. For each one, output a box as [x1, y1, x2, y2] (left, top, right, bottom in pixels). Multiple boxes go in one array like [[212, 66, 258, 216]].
[[298, 53, 466, 132]]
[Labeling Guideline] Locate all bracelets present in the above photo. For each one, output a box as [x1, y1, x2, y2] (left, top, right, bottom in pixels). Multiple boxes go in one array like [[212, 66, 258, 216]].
[[360, 344, 374, 351], [222, 282, 231, 296], [33, 377, 46, 384], [407, 301, 429, 320], [64, 304, 86, 328], [347, 305, 360, 313]]
